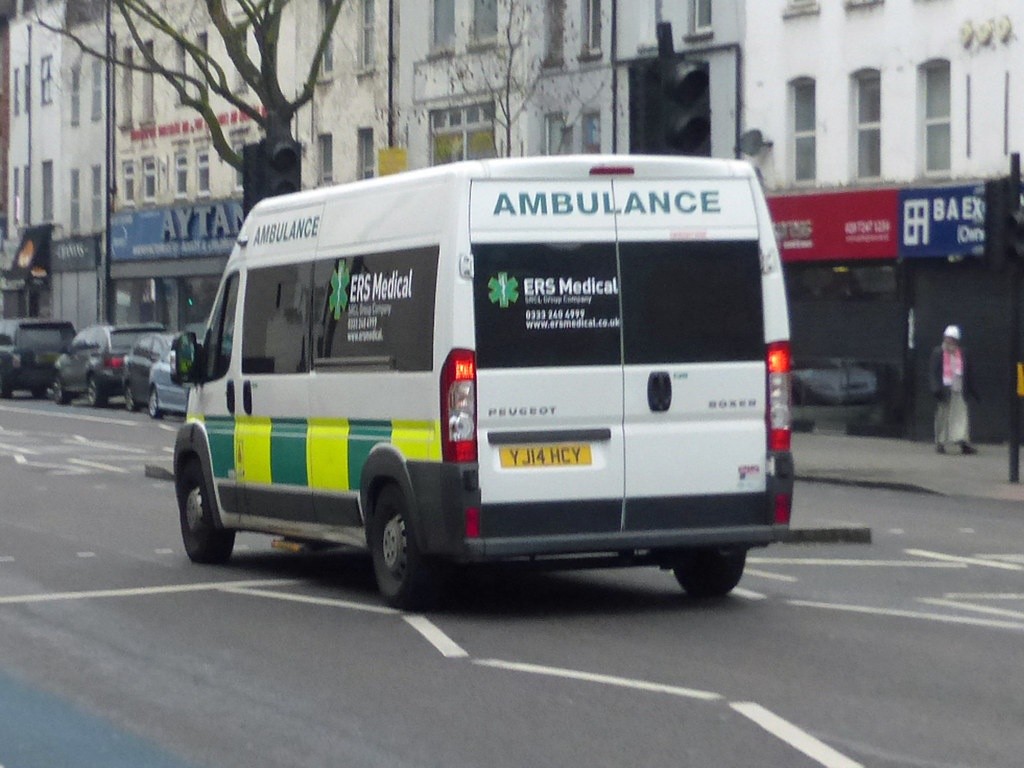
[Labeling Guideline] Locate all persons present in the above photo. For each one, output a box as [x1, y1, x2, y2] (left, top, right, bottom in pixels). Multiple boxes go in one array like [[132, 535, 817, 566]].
[[928, 325, 978, 455]]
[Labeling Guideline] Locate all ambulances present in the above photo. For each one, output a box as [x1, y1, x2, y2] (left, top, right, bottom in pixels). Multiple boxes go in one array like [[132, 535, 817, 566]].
[[173, 153, 794, 609]]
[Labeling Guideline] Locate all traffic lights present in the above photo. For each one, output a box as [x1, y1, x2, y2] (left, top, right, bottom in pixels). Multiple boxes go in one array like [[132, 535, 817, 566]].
[[244, 137, 301, 197], [628, 56, 711, 158]]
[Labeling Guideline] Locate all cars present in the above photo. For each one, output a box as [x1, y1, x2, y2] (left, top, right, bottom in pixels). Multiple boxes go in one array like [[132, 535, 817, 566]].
[[147, 339, 202, 420], [121, 333, 168, 412]]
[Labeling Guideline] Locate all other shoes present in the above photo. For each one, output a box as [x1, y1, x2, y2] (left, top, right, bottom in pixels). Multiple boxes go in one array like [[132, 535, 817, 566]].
[[961, 445, 979, 455], [937, 445, 945, 455]]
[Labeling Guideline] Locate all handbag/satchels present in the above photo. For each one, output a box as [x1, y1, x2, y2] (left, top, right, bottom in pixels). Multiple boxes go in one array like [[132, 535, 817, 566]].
[[933, 386, 952, 404]]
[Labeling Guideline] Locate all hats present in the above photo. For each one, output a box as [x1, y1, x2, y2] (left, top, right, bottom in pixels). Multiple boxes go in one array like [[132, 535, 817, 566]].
[[944, 325, 961, 340]]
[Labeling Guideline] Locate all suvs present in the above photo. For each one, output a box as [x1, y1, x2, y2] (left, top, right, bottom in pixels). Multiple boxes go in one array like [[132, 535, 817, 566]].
[[0, 318, 77, 399], [53, 323, 165, 408]]
